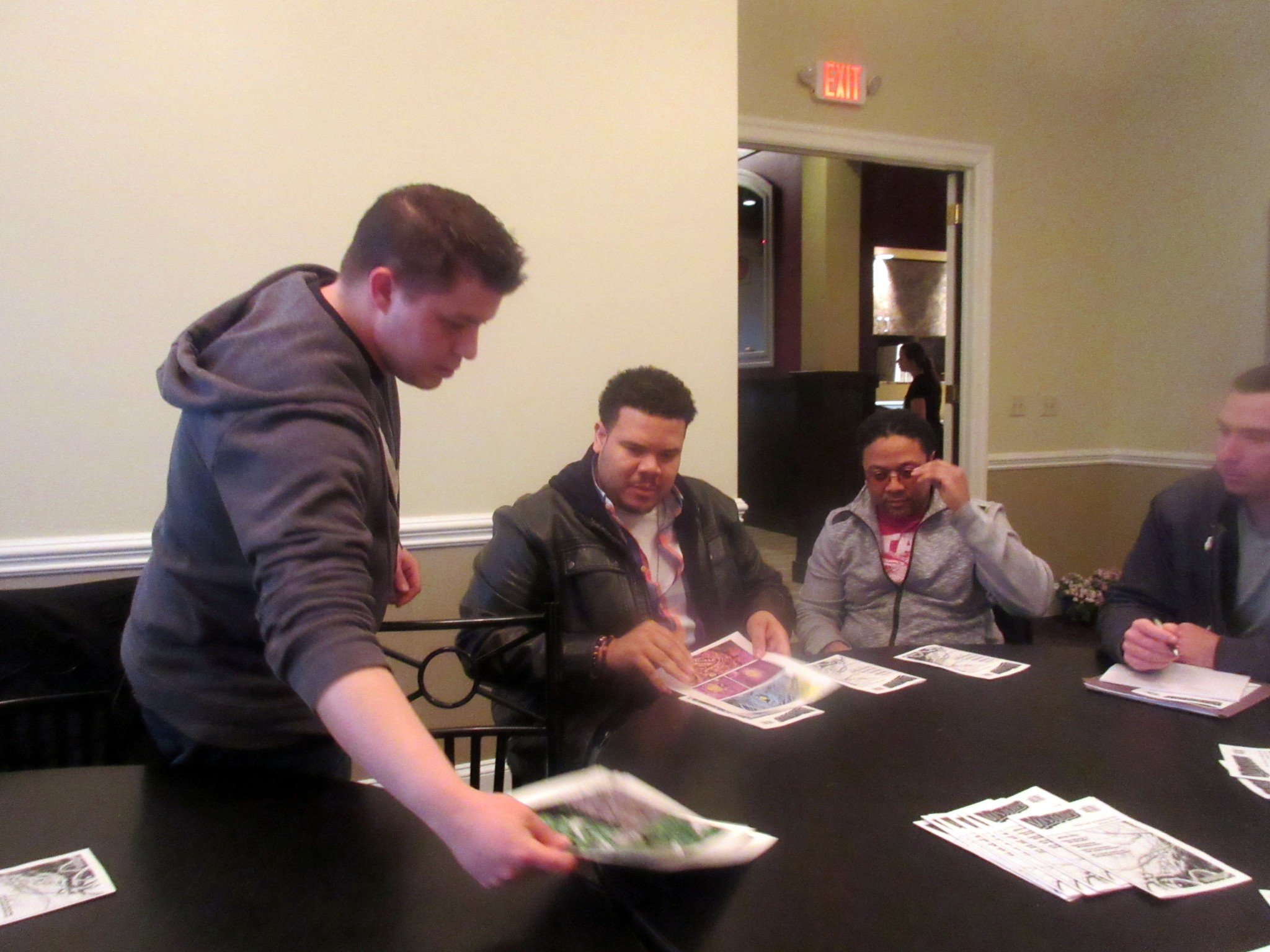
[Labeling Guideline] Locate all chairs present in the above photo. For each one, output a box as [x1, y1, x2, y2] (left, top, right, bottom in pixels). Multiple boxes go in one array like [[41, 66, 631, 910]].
[[0, 575, 149, 771], [377, 605, 562, 794]]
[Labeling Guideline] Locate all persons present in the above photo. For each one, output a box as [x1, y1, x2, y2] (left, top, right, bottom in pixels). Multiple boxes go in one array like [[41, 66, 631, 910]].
[[1097, 366, 1270, 684], [120, 183, 575, 888], [896, 342, 943, 459], [455, 364, 796, 786], [793, 409, 1057, 654]]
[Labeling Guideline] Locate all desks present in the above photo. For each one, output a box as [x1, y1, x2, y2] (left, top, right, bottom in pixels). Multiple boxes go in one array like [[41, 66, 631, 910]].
[[583, 634, 1270, 952], [0, 762, 573, 952]]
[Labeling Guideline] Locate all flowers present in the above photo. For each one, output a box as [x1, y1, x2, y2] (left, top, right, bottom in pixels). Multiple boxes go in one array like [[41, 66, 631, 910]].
[[1054, 562, 1121, 624]]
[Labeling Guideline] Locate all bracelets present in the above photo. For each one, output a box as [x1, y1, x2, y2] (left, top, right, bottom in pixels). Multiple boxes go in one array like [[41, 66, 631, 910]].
[[592, 634, 615, 674]]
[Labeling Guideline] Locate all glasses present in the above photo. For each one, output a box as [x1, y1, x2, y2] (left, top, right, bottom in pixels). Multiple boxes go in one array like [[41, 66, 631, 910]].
[[865, 467, 916, 489]]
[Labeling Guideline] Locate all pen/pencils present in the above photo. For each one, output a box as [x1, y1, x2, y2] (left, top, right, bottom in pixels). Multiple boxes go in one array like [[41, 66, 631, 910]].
[[1154, 619, 1178, 656]]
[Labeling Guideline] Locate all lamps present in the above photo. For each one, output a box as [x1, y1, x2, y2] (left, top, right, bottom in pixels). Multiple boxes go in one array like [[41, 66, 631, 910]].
[[799, 58, 882, 108]]
